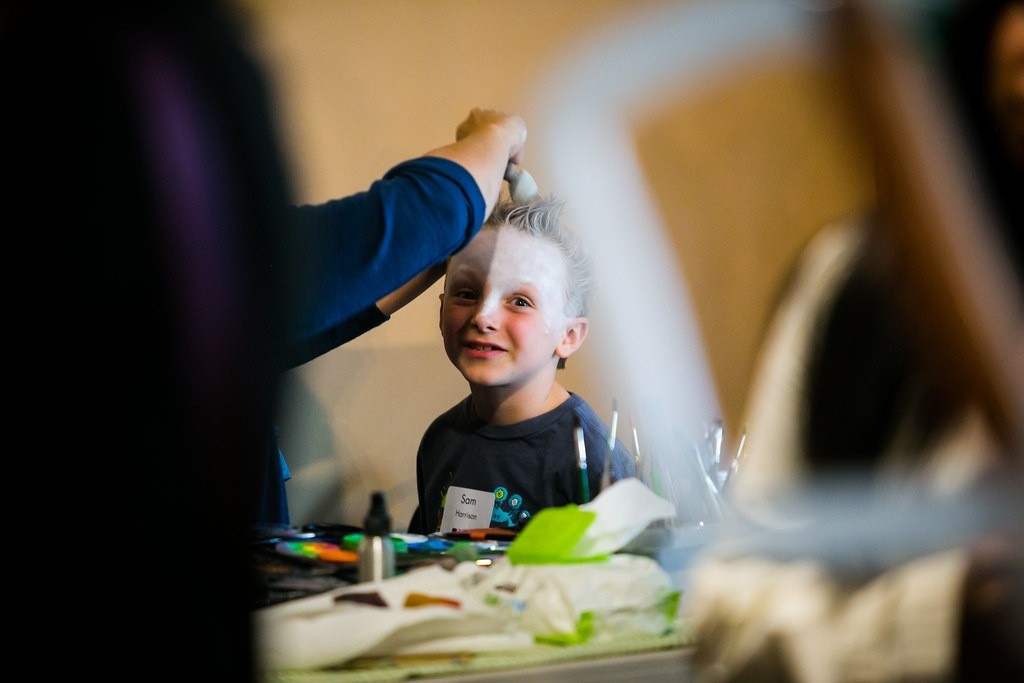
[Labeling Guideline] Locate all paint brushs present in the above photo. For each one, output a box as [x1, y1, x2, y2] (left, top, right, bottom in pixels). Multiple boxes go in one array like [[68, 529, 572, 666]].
[[572, 416, 590, 505], [600, 397, 619, 491], [627, 412, 747, 526]]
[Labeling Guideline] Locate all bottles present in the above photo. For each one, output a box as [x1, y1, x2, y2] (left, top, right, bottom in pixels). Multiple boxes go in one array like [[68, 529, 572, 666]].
[[359, 491, 395, 585]]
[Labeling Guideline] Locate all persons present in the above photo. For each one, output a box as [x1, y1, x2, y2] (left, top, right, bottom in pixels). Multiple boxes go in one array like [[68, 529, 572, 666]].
[[0, 0, 530, 683], [692, 0, 1024, 683], [405, 197, 675, 556]]
